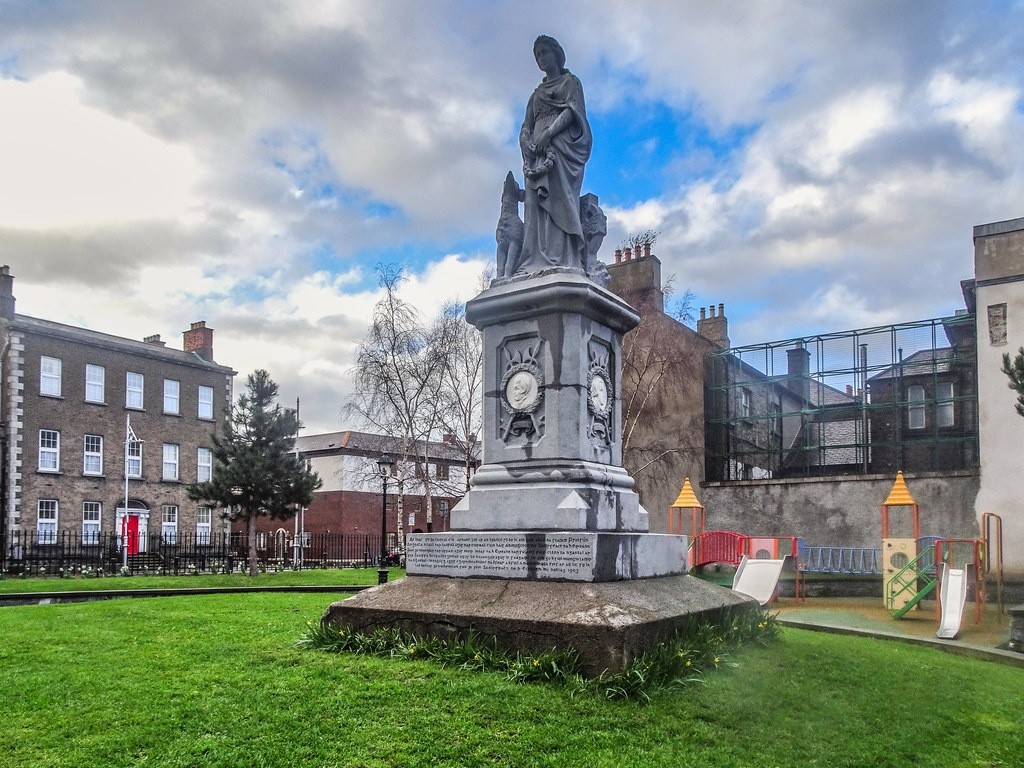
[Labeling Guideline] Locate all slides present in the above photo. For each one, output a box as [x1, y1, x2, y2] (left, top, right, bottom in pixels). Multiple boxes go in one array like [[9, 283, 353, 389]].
[[732, 553, 793, 606], [935, 562, 973, 639]]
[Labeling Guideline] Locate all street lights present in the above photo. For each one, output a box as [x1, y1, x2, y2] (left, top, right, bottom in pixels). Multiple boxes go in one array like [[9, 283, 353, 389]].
[[123, 439, 145, 572], [376, 451, 394, 584]]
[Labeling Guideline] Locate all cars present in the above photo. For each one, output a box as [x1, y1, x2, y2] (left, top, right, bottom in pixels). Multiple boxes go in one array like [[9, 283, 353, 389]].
[[379, 545, 400, 567]]
